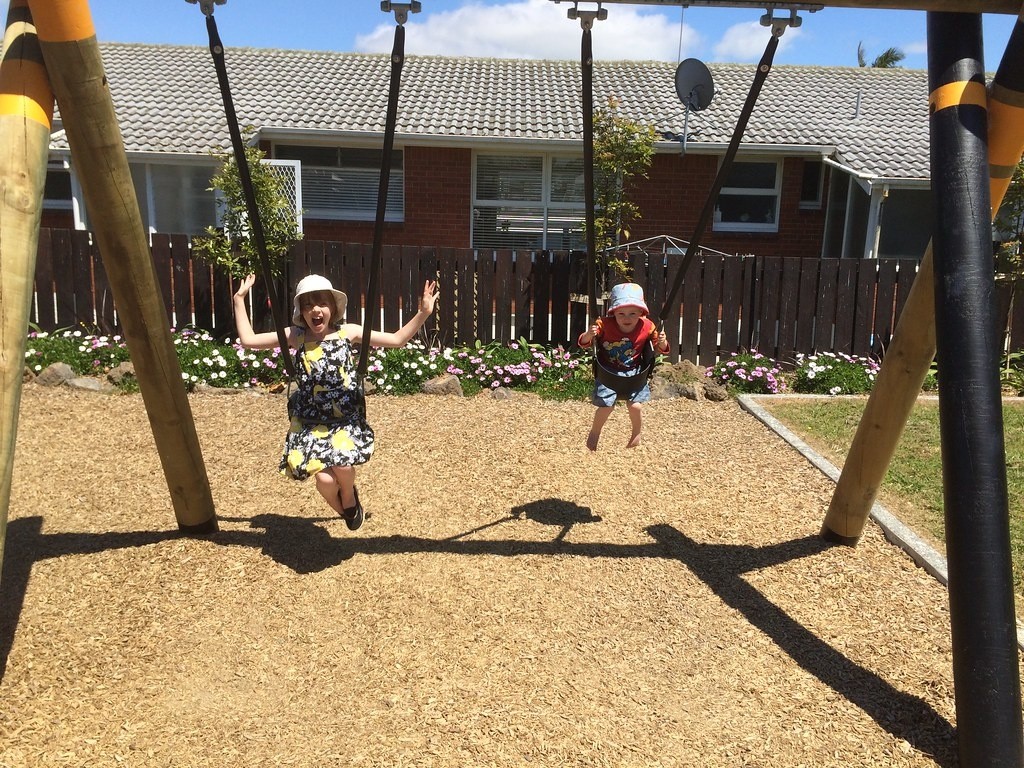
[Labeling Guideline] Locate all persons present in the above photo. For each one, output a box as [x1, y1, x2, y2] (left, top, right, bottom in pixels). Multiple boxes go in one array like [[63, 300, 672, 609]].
[[579, 284, 671, 452], [234, 271, 442, 531]]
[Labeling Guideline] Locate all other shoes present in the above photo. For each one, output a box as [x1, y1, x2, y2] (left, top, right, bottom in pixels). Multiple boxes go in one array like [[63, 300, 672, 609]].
[[338, 485, 364, 531]]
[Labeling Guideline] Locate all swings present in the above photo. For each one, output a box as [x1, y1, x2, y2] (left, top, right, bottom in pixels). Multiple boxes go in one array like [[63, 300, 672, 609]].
[[184, 1, 422, 431], [568, 3, 803, 399]]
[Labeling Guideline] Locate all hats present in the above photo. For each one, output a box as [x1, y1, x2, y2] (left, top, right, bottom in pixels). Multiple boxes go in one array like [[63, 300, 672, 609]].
[[292, 274, 348, 329], [607, 283, 650, 317]]
